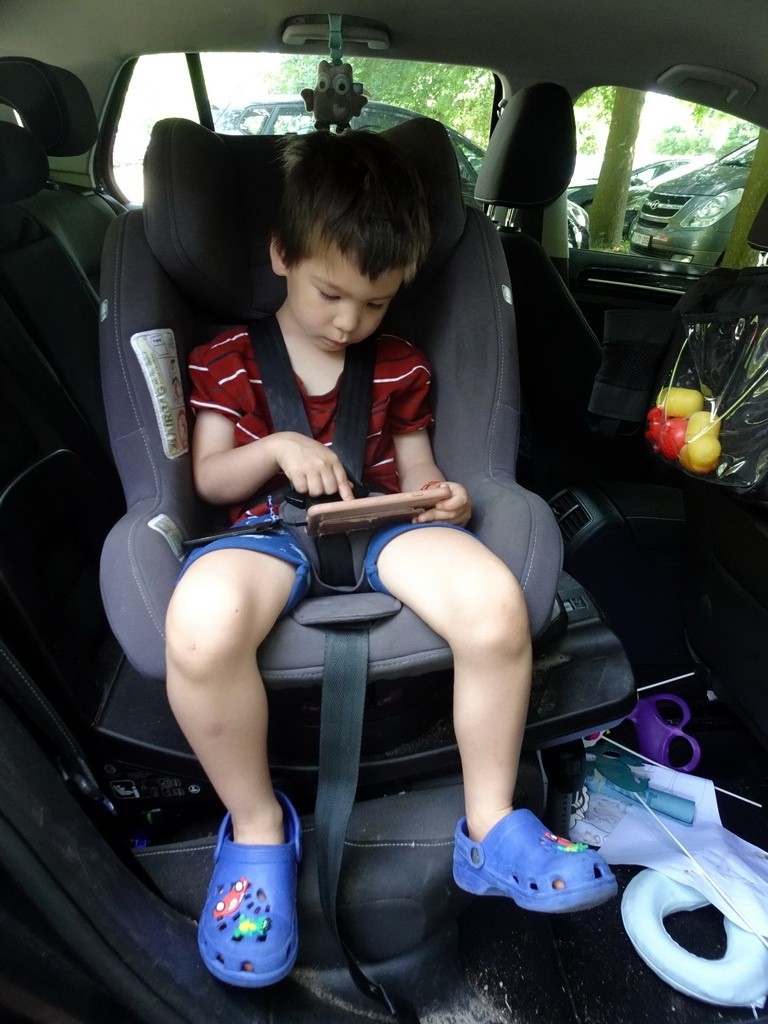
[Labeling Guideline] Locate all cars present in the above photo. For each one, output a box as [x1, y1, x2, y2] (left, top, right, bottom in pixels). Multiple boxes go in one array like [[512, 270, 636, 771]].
[[214, 88, 591, 252], [625, 131, 768, 266], [567, 152, 695, 237]]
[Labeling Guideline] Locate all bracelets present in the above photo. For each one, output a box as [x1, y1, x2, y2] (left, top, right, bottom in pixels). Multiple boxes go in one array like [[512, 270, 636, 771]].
[[421, 481, 441, 491]]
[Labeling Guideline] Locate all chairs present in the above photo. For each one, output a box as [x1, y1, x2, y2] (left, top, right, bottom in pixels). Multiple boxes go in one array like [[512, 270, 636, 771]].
[[0, 121, 643, 817], [98, 120, 562, 679], [668, 193, 768, 748], [472, 80, 659, 496], [0, 56, 127, 452]]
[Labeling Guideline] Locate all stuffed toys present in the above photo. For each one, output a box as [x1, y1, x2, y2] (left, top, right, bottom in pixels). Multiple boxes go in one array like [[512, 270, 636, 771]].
[[300, 60, 367, 133]]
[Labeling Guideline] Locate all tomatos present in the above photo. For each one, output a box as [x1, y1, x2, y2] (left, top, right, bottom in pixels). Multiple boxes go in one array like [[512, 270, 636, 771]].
[[647, 388, 721, 473]]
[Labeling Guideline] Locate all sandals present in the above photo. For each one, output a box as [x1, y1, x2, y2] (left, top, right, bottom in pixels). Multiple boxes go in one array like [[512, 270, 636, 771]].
[[452, 809, 619, 915], [197, 791, 305, 988]]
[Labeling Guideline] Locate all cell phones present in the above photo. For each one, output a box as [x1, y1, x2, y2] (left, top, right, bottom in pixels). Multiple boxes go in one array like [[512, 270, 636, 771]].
[[304, 487, 451, 537]]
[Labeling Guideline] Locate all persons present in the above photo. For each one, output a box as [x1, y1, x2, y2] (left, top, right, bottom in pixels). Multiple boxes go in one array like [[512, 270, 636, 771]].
[[165, 130, 617, 988]]
